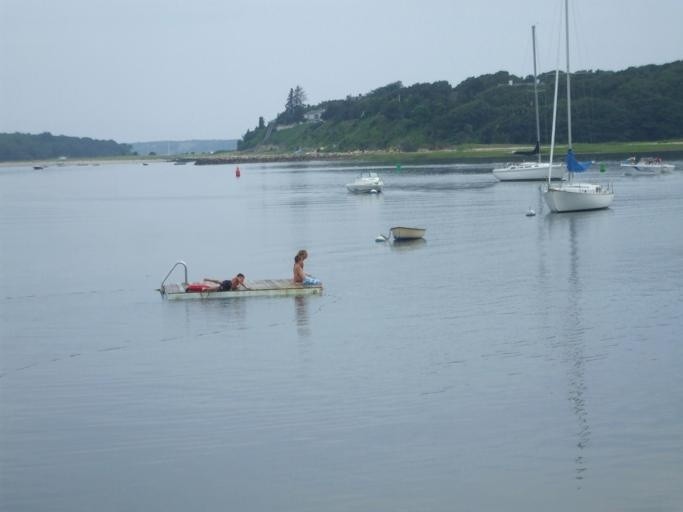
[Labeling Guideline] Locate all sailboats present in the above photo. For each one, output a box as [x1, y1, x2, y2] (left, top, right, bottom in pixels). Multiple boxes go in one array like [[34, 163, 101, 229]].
[[542, 0, 615, 211]]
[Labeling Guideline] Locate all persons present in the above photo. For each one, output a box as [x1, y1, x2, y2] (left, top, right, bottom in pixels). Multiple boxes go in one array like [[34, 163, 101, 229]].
[[202, 273, 253, 292], [293, 255, 305, 282], [297, 250, 310, 277]]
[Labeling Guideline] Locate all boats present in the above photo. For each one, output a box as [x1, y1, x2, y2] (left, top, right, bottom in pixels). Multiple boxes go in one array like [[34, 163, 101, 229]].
[[346, 172, 383, 193], [159, 259, 325, 299], [376, 226, 426, 241], [618, 157, 675, 175], [493, 26, 567, 181]]
